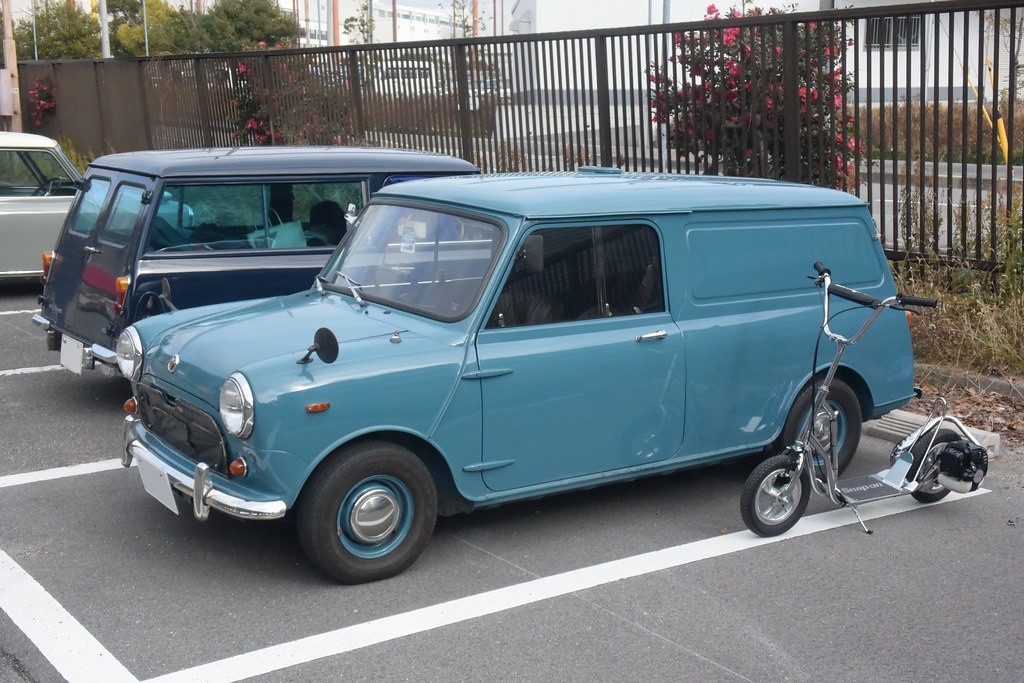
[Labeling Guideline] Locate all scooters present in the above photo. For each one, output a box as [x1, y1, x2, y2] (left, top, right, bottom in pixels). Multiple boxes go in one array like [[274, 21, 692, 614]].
[[739, 261, 989, 537]]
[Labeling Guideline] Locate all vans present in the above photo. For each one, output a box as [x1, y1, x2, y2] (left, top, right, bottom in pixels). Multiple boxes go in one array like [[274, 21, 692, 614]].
[[114, 167, 925, 584], [31, 150, 495, 378], [360, 61, 436, 95]]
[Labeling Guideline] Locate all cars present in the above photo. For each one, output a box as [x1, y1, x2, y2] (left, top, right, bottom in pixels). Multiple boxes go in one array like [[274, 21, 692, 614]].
[[467, 78, 513, 99], [0, 131, 192, 279]]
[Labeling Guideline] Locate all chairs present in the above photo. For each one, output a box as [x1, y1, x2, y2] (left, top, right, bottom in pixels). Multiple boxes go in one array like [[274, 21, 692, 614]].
[[310, 201, 348, 246], [525, 293, 565, 327]]
